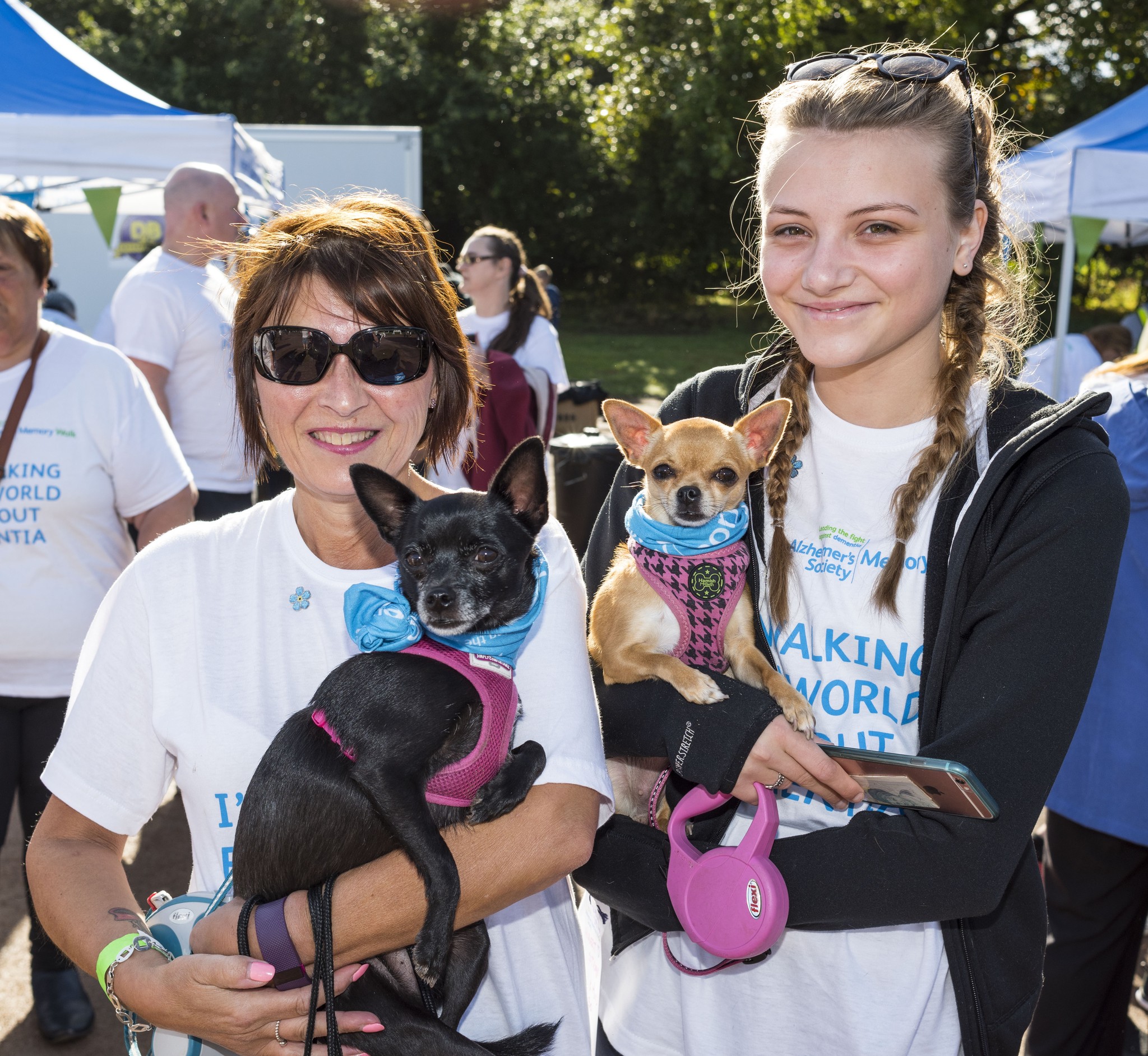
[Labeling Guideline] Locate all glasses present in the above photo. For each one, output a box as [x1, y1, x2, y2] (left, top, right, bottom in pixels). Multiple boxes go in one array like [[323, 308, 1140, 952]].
[[252, 325, 431, 386], [786, 52, 979, 187], [457, 253, 494, 266]]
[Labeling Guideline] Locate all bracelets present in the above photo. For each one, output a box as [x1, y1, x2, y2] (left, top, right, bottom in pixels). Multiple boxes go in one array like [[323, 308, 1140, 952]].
[[255, 895, 313, 991], [96, 930, 174, 1033]]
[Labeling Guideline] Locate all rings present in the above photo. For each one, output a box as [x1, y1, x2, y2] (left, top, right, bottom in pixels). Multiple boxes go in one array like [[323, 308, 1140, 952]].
[[275, 1020, 287, 1047], [764, 773, 784, 790]]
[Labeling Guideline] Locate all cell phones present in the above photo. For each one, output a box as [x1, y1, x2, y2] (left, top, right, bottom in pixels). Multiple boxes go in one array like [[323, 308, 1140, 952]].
[[816, 741, 1001, 820]]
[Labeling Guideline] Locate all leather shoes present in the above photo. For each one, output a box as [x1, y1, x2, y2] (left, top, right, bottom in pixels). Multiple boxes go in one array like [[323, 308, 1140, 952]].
[[33, 965, 95, 1042]]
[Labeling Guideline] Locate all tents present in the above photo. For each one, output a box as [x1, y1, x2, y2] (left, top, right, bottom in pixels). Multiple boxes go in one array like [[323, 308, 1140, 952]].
[[989, 84, 1148, 403], [0, 0, 287, 279]]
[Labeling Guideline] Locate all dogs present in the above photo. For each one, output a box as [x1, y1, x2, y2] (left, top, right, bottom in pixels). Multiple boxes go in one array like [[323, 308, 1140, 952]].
[[588, 398, 816, 836], [232, 435, 564, 1056]]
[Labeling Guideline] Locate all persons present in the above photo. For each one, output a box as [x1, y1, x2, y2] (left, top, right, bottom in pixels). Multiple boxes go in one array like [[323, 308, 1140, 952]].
[[1017, 321, 1148, 1056], [0, 196, 200, 1046], [417, 223, 570, 492], [109, 161, 268, 521], [569, 40, 1129, 1056], [19, 193, 613, 1056]]
